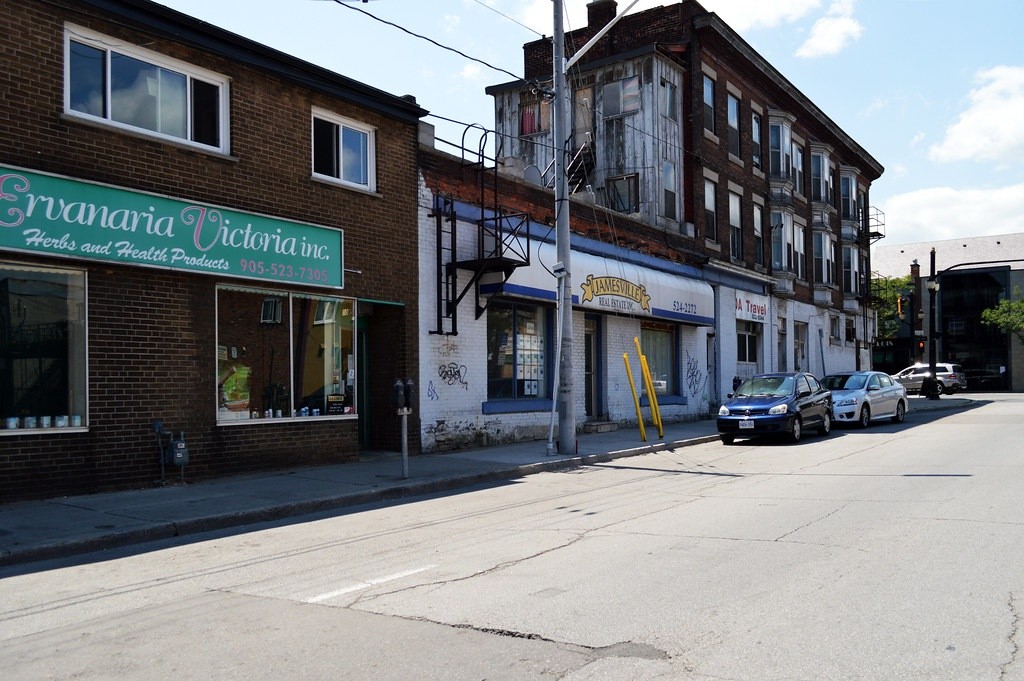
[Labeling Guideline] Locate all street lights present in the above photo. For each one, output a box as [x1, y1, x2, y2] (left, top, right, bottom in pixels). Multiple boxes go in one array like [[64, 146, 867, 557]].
[[925, 247, 944, 400], [897, 290, 926, 367]]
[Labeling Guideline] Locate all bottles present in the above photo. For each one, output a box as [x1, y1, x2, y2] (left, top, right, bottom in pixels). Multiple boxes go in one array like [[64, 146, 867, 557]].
[[251, 406, 320, 419], [5, 415, 82, 429]]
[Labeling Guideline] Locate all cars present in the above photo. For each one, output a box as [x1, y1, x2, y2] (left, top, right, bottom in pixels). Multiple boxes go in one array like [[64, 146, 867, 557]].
[[717, 371, 835, 444], [818, 370, 910, 427]]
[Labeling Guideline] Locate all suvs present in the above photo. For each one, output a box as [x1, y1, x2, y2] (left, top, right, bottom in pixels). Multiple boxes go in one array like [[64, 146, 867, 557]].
[[889, 361, 967, 396]]
